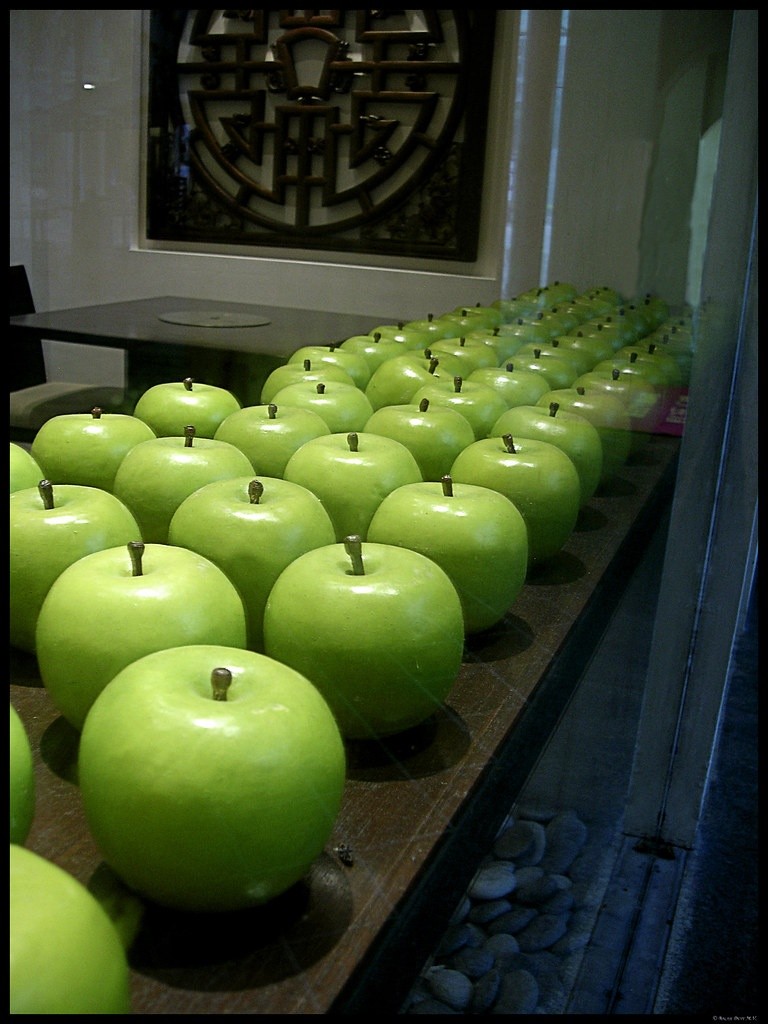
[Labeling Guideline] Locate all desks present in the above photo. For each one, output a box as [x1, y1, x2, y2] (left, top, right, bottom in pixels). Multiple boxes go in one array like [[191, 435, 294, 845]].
[[9, 296, 417, 407]]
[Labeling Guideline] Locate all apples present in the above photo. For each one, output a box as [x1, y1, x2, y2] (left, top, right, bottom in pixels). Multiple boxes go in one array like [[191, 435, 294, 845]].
[[9, 280, 691, 1013]]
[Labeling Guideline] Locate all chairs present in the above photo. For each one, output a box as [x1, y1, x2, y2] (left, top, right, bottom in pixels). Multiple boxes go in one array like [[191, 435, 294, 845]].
[[9, 265, 125, 445]]
